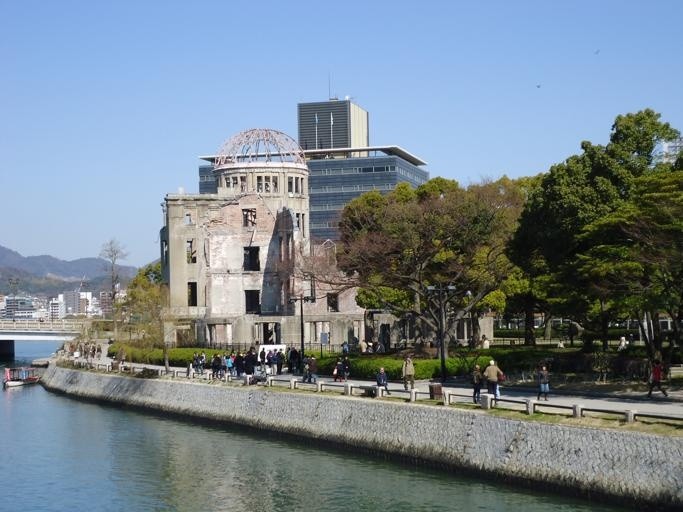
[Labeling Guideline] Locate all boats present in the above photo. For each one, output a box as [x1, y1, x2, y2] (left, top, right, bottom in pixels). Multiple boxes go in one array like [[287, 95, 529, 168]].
[[4, 366, 41, 387]]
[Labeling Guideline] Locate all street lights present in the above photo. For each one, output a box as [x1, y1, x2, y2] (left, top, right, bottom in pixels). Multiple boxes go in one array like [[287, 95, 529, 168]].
[[288, 296, 314, 357], [426, 281, 459, 383]]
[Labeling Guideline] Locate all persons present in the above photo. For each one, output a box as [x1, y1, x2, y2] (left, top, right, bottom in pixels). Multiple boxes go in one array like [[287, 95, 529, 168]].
[[361, 339, 385, 355], [472, 359, 504, 404], [191, 347, 317, 384], [115, 347, 127, 365], [557, 338, 563, 348], [643, 359, 669, 400], [619, 334, 634, 349], [538, 364, 550, 401], [67, 340, 103, 361], [332, 341, 350, 382], [376, 367, 391, 396], [399, 355, 415, 391], [468, 333, 490, 349]]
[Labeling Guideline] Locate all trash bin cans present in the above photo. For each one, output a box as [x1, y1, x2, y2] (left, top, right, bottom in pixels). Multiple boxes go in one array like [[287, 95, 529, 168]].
[[429, 383, 442, 400]]
